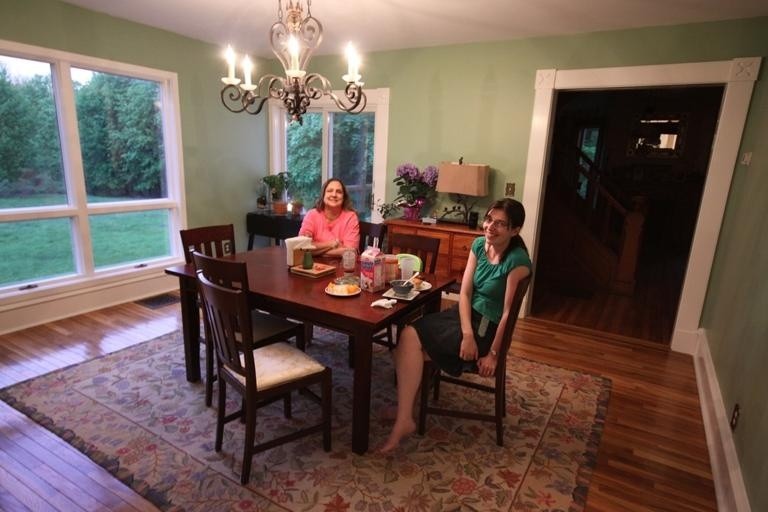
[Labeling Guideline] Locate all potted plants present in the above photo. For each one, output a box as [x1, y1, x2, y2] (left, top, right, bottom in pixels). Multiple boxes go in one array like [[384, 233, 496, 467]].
[[291, 198, 304, 216], [263, 171, 297, 214]]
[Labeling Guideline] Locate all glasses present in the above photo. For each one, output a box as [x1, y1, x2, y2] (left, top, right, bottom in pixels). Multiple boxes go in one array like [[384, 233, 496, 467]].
[[483, 215, 507, 228]]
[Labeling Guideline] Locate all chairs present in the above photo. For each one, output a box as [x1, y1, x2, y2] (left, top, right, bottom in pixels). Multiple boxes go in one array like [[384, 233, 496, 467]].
[[180, 224, 235, 344], [418, 270, 530, 446], [193, 251, 306, 424], [349, 221, 386, 368], [372, 232, 440, 387], [196, 269, 331, 484]]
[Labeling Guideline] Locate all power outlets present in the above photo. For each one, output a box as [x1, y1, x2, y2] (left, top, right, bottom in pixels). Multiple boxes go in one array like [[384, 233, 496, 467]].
[[730, 404, 740, 430]]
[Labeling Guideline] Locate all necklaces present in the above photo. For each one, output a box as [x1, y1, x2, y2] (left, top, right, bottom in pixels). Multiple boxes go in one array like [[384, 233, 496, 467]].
[[324, 211, 341, 221]]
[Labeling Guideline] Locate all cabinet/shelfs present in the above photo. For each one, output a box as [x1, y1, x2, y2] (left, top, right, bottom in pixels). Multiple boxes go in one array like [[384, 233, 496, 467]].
[[383, 217, 486, 302]]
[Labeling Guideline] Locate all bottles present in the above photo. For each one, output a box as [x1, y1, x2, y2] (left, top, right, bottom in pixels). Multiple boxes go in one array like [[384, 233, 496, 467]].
[[303, 251, 314, 269], [383, 254, 399, 284], [256, 179, 269, 215]]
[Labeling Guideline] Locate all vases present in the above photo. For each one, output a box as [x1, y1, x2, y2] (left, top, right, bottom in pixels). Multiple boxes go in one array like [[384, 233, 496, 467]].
[[400, 206, 423, 221]]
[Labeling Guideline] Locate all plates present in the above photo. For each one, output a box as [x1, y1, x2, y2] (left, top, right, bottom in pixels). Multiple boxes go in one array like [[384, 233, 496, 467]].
[[404, 279, 433, 292], [382, 288, 421, 302], [325, 283, 362, 298]]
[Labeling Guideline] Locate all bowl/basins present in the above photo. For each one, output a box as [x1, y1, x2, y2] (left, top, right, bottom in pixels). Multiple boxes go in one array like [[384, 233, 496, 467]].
[[390, 280, 414, 297], [333, 275, 360, 285]]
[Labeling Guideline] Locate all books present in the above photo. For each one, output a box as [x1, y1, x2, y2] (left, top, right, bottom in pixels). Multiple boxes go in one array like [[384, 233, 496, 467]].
[[287, 261, 337, 279]]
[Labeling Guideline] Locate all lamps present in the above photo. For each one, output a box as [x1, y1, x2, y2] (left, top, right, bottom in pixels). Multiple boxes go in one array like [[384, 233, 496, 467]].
[[220, 0, 366, 125], [435, 161, 490, 223]]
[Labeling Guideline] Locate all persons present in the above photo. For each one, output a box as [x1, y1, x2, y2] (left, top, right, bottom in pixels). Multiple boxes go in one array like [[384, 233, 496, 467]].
[[378, 198, 533, 455], [298, 177, 361, 259]]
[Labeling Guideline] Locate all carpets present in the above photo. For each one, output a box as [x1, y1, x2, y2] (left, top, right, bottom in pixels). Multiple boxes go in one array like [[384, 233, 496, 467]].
[[0, 308, 612, 512]]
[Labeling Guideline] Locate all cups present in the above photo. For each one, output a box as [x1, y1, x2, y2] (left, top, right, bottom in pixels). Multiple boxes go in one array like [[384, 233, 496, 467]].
[[401, 258, 415, 280], [343, 248, 356, 277]]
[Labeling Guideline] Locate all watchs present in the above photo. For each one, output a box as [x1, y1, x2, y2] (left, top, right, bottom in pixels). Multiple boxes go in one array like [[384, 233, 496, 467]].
[[489, 347, 499, 357]]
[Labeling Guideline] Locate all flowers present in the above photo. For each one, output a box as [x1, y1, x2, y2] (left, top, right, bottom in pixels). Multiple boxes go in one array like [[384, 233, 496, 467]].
[[393, 163, 438, 206]]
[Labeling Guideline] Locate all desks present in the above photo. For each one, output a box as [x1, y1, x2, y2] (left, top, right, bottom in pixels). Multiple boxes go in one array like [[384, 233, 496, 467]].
[[247, 210, 304, 251]]
